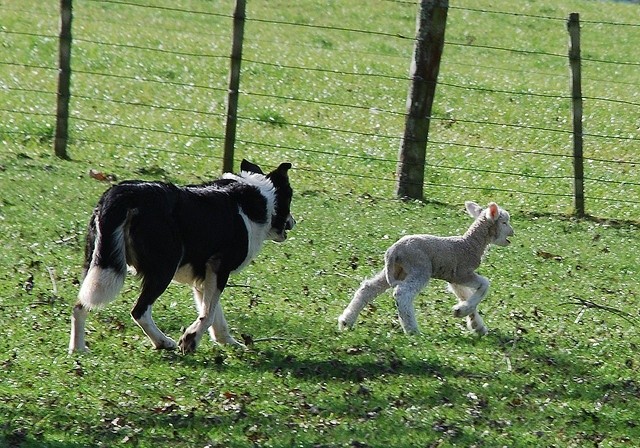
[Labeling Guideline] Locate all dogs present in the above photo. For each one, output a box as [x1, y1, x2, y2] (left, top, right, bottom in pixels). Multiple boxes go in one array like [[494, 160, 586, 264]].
[[69, 158, 297, 356]]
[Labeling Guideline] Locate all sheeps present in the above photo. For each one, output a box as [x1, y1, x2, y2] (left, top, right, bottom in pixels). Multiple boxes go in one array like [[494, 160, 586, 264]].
[[338, 200, 514, 336]]
